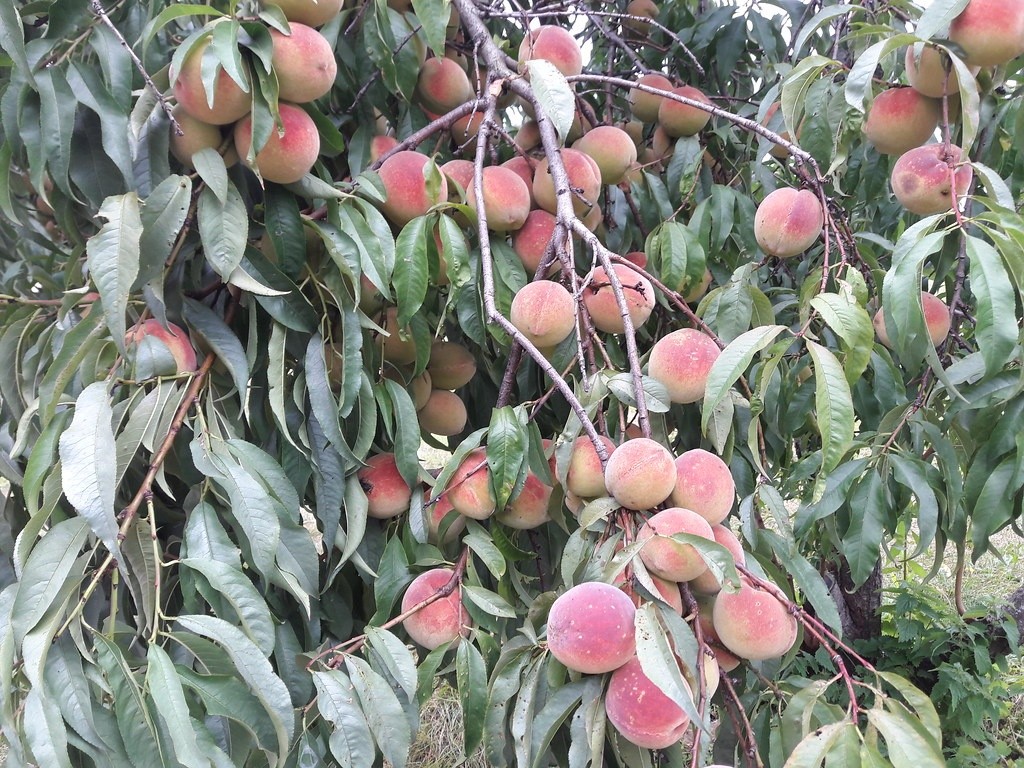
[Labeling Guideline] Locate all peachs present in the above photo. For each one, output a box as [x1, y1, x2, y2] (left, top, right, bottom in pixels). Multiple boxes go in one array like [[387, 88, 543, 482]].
[[121, 0, 1024, 749]]
